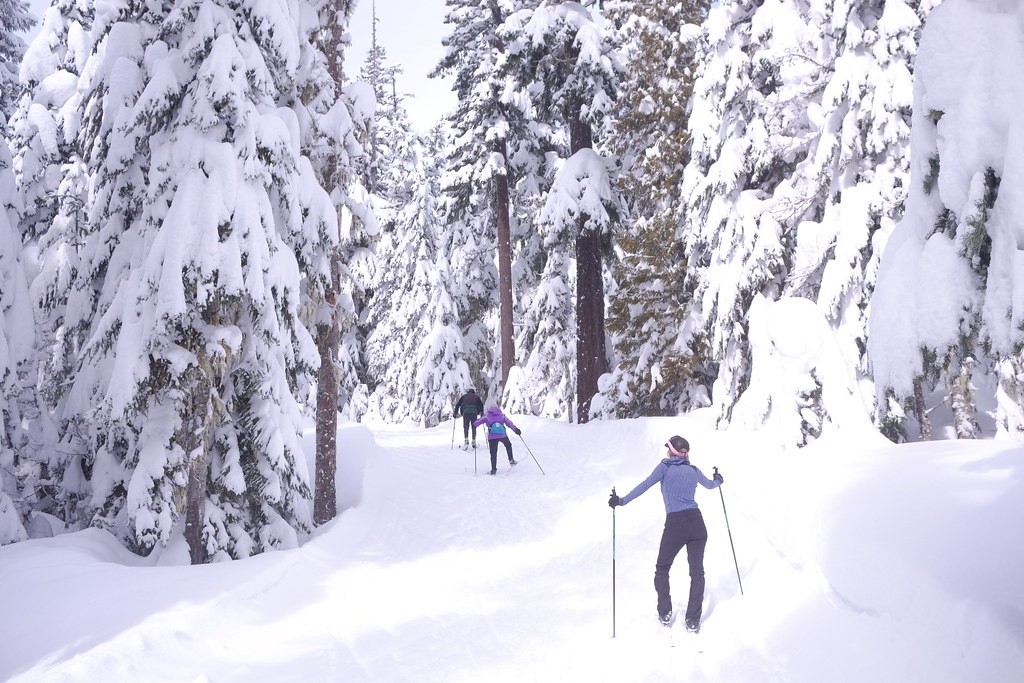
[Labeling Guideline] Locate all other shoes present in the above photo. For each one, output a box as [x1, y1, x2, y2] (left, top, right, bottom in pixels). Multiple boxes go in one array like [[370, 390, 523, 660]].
[[490, 469, 496, 474], [472, 439, 476, 447], [510, 459, 518, 465], [464, 440, 469, 444], [660, 610, 672, 623], [685, 615, 700, 631]]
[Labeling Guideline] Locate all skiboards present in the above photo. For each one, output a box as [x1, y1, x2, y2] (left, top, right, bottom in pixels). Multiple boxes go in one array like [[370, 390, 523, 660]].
[[458, 444, 481, 452], [486, 453, 530, 476]]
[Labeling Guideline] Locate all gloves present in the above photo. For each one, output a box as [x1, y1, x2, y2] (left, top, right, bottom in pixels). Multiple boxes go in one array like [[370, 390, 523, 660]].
[[514, 428, 521, 435], [713, 474, 723, 484], [453, 413, 458, 418], [609, 495, 619, 508]]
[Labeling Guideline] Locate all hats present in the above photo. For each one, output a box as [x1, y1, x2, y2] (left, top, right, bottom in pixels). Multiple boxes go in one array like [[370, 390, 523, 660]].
[[665, 436, 689, 456]]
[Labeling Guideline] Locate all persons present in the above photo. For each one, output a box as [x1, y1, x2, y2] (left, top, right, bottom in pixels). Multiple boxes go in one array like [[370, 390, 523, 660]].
[[453, 389, 483, 449], [473, 404, 521, 474], [609, 436, 723, 632]]
